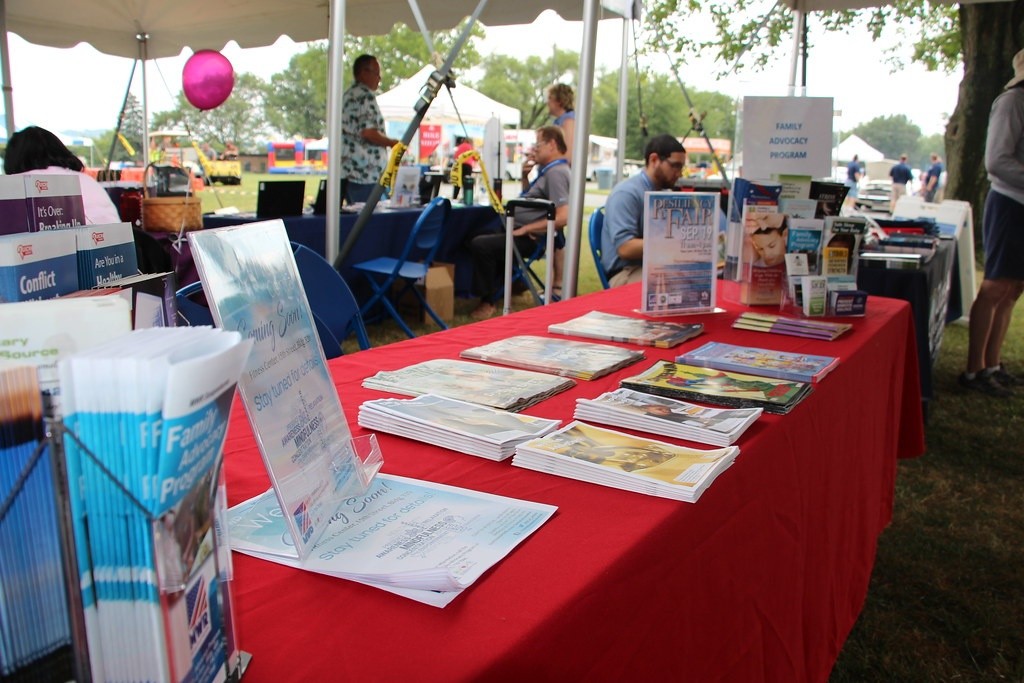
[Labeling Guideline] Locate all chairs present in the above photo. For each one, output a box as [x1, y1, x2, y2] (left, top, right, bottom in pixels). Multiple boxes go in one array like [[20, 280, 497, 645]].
[[151, 280, 343, 367], [589, 206, 610, 289], [346, 196, 452, 339], [283, 242, 370, 351], [487, 233, 561, 306]]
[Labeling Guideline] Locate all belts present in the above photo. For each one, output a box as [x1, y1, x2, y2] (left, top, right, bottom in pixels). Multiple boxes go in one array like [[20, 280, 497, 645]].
[[607, 267, 623, 283]]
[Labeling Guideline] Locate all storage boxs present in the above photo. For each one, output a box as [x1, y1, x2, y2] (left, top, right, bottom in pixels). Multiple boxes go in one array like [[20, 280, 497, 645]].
[[396, 261, 456, 325]]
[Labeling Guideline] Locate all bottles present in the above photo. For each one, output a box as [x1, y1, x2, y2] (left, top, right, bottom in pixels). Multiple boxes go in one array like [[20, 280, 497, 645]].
[[464, 175, 475, 206]]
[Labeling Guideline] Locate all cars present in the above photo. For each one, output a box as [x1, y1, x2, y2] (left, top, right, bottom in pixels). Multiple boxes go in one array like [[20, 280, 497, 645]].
[[856, 159, 901, 209]]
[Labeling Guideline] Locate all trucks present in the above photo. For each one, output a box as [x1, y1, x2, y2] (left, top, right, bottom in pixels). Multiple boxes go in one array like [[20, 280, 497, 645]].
[[500, 128, 538, 181], [586, 134, 618, 182]]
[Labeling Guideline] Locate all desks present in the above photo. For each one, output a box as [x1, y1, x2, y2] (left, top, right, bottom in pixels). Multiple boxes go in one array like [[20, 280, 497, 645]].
[[221, 276, 924, 683], [198, 194, 512, 320], [856, 200, 976, 391]]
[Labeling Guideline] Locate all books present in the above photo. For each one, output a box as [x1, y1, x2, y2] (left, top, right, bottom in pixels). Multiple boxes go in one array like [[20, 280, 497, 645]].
[[358, 310, 852, 504], [744, 212, 789, 305], [820, 216, 867, 277], [860, 233, 940, 271], [222, 470, 559, 609]]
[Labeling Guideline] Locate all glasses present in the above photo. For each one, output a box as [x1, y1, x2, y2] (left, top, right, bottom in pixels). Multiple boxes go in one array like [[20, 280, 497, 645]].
[[531, 141, 546, 147], [661, 155, 686, 174]]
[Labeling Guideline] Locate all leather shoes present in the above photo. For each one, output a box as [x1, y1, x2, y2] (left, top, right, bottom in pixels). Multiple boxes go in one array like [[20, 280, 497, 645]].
[[471, 303, 496, 319]]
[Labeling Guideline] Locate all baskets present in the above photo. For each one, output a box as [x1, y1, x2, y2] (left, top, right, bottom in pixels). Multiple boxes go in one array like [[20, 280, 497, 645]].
[[141, 159, 203, 231]]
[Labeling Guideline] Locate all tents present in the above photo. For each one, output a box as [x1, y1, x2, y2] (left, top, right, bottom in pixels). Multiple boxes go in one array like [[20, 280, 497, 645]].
[[0, 0, 640, 302], [372, 64, 522, 183], [832, 134, 883, 166]]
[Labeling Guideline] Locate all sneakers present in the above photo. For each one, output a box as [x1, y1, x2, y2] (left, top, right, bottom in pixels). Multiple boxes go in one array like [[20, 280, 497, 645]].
[[960, 362, 1024, 397]]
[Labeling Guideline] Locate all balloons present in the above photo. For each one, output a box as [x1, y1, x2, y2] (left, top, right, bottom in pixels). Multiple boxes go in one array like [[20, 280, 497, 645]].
[[182, 50, 235, 112]]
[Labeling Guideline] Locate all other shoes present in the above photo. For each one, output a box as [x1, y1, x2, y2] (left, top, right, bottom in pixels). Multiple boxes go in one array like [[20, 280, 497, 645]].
[[539, 286, 562, 303]]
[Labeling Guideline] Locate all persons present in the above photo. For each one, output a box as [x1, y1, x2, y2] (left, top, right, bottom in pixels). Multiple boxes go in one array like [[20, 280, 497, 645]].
[[828, 235, 856, 273], [749, 213, 788, 267], [471, 84, 580, 301], [453, 137, 478, 199], [3, 124, 122, 230], [342, 51, 399, 206], [960, 50, 1024, 397], [600, 132, 687, 290], [844, 154, 942, 213]]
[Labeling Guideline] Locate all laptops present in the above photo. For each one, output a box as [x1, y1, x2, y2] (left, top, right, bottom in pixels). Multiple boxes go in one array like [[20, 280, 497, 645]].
[[419, 173, 444, 205], [255, 181, 305, 220], [312, 179, 349, 214]]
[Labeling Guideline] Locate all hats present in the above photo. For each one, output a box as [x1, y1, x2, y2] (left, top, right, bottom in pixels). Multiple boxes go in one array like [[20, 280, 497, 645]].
[[1004, 49, 1024, 90]]
